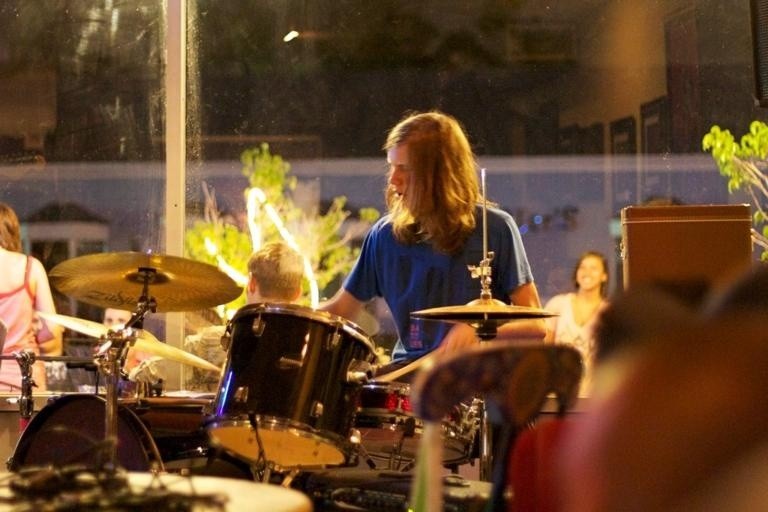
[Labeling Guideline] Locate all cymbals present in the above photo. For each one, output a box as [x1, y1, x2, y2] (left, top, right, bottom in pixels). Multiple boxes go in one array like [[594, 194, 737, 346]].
[[409, 296, 558, 327], [49, 252, 244, 313], [34, 310, 222, 371]]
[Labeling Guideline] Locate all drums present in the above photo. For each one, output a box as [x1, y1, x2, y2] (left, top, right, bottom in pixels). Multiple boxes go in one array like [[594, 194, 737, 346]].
[[1, 470, 314, 512], [281, 468, 513, 511], [202, 303, 380, 470], [7, 394, 166, 477], [354, 380, 482, 465]]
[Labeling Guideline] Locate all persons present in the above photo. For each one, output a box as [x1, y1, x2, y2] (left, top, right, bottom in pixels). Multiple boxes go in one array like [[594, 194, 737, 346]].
[[183, 240, 305, 392], [0, 201, 63, 392], [103, 308, 132, 326], [543, 251, 610, 365], [313, 111, 541, 376]]
